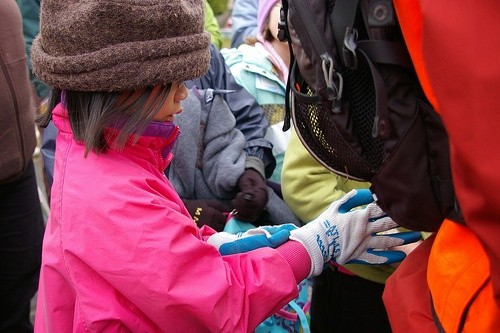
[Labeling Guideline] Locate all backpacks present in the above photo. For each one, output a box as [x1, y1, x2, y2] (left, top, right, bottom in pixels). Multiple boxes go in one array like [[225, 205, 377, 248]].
[[277, 0, 469, 232]]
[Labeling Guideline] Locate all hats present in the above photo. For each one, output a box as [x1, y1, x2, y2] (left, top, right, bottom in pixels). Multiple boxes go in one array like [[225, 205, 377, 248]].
[[31, 0, 211, 91], [258, 0, 279, 34]]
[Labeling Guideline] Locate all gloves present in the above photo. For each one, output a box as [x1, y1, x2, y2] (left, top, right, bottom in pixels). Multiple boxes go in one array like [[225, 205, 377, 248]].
[[184, 199, 226, 232], [206, 223, 298, 256], [289, 189, 423, 279], [230, 169, 269, 223]]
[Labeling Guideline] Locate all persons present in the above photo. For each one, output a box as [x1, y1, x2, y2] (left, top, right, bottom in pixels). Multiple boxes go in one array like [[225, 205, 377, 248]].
[[0, 1, 433, 333], [381, 0, 500, 333]]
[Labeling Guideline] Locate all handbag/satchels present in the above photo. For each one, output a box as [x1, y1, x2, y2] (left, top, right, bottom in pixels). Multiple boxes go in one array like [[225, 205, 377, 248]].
[[223, 209, 312, 333]]
